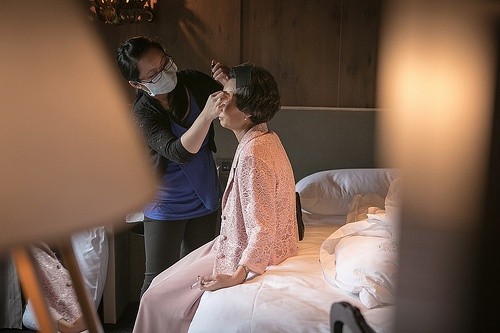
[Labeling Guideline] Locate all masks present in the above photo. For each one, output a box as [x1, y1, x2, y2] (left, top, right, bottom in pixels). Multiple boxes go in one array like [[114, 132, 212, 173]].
[[139, 62, 178, 97]]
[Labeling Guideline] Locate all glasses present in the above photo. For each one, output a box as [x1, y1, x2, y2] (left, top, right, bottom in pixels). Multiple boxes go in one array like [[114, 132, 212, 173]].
[[137, 54, 174, 84]]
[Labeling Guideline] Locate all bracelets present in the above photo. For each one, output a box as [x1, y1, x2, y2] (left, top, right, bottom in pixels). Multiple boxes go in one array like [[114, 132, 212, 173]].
[[236, 264, 248, 284]]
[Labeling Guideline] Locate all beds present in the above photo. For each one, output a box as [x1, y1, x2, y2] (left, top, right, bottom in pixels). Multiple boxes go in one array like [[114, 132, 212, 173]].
[[187, 209, 396, 333]]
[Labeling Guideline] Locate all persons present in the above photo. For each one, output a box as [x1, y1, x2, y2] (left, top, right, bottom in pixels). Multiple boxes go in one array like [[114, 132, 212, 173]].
[[132, 64, 300, 333], [22, 224, 108, 333], [116, 37, 230, 298]]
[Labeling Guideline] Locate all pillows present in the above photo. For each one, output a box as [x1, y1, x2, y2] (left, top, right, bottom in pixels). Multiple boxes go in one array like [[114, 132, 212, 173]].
[[291, 166, 391, 216]]
